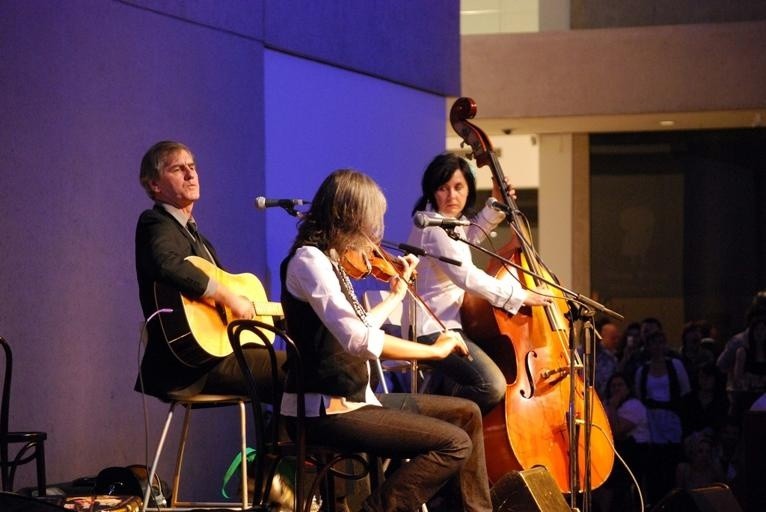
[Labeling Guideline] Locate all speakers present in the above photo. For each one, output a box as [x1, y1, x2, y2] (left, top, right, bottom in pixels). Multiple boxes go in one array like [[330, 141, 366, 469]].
[[490, 464, 573, 512], [664, 483, 743, 512]]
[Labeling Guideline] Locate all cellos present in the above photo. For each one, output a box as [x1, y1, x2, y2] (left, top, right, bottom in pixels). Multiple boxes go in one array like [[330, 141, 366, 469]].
[[450, 98, 615, 494]]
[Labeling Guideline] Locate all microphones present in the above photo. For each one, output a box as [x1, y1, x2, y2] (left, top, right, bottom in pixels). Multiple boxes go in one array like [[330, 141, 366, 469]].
[[486, 196, 519, 214], [256, 197, 303, 209], [414, 213, 470, 228]]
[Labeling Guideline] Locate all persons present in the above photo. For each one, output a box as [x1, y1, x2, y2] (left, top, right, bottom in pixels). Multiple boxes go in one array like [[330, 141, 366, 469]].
[[131, 137, 289, 404], [277, 170, 498, 511], [589, 292, 766, 512], [400, 152, 560, 422]]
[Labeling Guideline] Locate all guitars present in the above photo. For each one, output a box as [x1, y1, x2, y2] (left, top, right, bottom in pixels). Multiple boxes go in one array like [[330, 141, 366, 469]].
[[153, 255, 284, 369]]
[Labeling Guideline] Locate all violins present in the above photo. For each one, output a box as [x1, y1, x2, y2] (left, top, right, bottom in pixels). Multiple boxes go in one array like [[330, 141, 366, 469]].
[[342, 244, 419, 284]]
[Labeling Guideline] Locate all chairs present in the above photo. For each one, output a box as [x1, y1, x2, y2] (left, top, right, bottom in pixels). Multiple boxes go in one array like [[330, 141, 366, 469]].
[[138, 320, 255, 510], [0, 336, 47, 496], [225, 319, 381, 512]]
